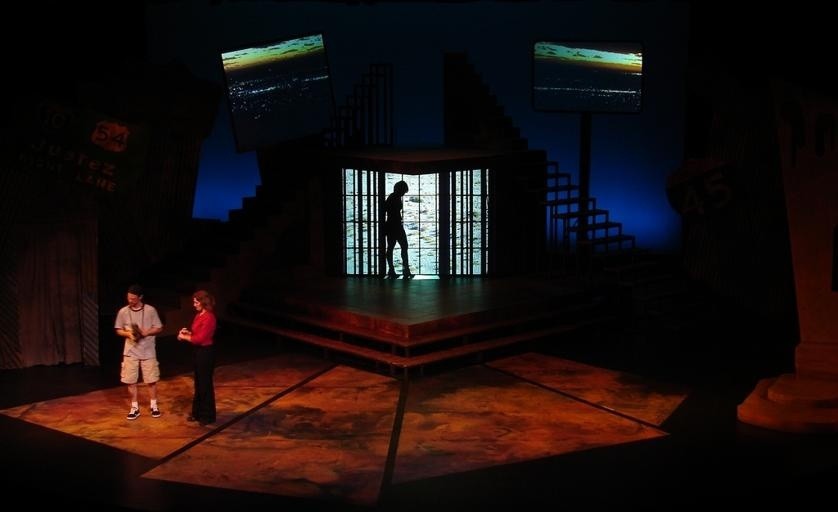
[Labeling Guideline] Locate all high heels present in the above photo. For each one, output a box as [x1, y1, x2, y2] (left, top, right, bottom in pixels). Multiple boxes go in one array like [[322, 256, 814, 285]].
[[388, 268, 397, 278], [402, 268, 414, 278]]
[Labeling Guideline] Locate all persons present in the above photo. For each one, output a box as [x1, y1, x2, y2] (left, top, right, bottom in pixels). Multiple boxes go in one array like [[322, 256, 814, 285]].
[[114, 285, 164, 420], [176, 290, 217, 424], [381, 181, 415, 279]]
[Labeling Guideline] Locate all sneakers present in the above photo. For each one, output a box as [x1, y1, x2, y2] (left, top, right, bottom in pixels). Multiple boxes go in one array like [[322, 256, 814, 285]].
[[151, 406, 160, 418], [126, 408, 141, 419]]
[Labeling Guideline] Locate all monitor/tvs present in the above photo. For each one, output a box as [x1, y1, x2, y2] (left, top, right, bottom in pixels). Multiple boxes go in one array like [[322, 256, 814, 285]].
[[528, 39, 650, 118], [215, 28, 340, 153]]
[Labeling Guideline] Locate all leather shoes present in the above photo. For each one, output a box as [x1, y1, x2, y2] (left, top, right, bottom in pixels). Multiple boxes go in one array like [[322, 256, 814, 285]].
[[187, 414, 216, 424]]
